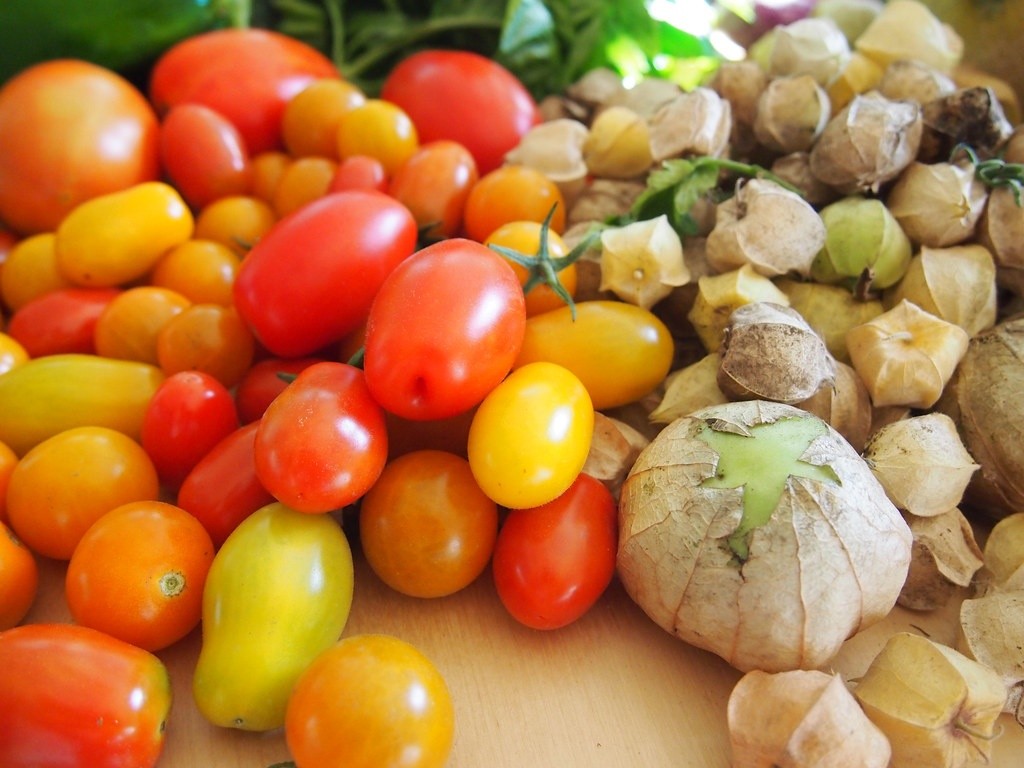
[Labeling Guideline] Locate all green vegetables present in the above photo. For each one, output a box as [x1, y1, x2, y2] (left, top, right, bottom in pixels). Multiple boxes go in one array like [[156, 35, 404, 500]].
[[0, 0, 731, 102]]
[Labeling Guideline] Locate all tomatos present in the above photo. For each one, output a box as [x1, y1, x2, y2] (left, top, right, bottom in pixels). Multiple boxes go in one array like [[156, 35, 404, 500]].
[[0, 28, 672, 768]]
